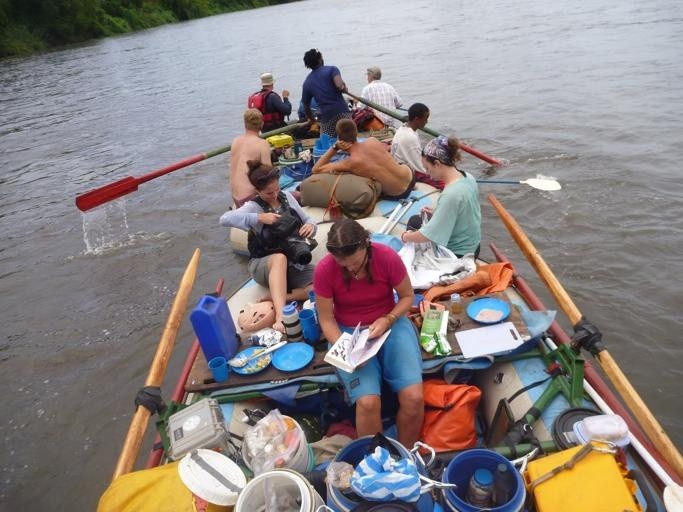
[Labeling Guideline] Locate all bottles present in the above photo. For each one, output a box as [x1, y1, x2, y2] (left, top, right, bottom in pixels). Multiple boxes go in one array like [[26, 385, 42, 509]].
[[492, 463, 514, 504], [282, 301, 301, 341], [281, 139, 302, 160], [463, 467, 493, 506], [450, 293, 460, 314]]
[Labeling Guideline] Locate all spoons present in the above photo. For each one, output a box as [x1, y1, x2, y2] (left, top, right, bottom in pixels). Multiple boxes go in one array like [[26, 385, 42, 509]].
[[227, 340, 287, 368]]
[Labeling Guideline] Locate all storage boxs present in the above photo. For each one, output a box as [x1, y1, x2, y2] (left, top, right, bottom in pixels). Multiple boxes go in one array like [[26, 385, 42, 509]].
[[522, 440, 642, 512], [167, 397, 232, 462]]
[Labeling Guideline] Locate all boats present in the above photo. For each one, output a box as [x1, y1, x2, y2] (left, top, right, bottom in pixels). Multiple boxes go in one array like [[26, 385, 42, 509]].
[[162, 255, 665, 511], [222, 136, 442, 268]]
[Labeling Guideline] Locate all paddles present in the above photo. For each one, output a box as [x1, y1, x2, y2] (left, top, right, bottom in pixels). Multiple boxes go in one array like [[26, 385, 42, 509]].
[[476, 176, 561, 191], [76, 122, 312, 212]]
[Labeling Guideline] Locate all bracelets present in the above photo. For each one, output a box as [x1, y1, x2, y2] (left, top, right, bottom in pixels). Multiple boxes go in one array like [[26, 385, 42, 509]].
[[401, 232, 408, 243], [384, 312, 400, 323]]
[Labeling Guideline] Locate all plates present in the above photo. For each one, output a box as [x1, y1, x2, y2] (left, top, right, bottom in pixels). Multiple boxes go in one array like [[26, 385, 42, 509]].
[[270, 342, 314, 372], [466, 297, 511, 325], [230, 346, 272, 376]]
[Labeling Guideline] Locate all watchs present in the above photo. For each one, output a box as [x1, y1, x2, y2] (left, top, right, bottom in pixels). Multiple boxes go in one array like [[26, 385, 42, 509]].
[[332, 142, 339, 150]]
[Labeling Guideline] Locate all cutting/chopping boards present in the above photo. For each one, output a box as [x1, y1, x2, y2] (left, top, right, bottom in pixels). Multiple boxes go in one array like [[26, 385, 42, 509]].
[[454, 322, 524, 359]]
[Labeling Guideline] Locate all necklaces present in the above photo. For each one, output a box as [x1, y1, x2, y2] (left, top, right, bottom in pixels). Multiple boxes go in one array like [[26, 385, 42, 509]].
[[353, 253, 367, 281]]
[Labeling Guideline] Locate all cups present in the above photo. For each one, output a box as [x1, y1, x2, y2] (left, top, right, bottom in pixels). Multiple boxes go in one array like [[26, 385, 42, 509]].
[[208, 357, 232, 383], [299, 309, 319, 342]]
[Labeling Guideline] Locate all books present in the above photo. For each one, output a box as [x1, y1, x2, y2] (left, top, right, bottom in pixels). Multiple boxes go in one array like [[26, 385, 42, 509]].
[[323, 321, 391, 374]]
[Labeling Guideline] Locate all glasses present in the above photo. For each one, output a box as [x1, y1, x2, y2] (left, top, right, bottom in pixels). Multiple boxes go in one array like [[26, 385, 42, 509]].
[[327, 240, 362, 256]]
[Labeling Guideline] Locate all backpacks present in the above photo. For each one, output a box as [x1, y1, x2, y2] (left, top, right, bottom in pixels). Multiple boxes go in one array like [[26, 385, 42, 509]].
[[256, 207, 299, 256]]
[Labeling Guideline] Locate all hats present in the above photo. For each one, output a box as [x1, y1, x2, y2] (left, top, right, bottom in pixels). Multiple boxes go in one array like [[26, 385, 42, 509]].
[[260, 73, 274, 86]]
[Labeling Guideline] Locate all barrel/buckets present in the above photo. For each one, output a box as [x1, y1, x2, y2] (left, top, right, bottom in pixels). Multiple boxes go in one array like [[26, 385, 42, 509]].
[[232, 467, 325, 512], [241, 414, 312, 474], [324, 433, 436, 512], [439, 447, 527, 512], [189, 292, 239, 361], [312, 145, 348, 165], [277, 149, 313, 182]]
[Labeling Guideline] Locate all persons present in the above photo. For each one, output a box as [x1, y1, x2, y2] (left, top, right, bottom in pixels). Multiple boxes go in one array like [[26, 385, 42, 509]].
[[356, 66, 403, 127], [229, 108, 301, 208], [248, 73, 292, 134], [301, 48, 352, 137], [311, 118, 416, 201], [400, 135, 482, 276], [219, 159, 317, 333], [391, 103, 445, 190], [313, 218, 425, 449]]
